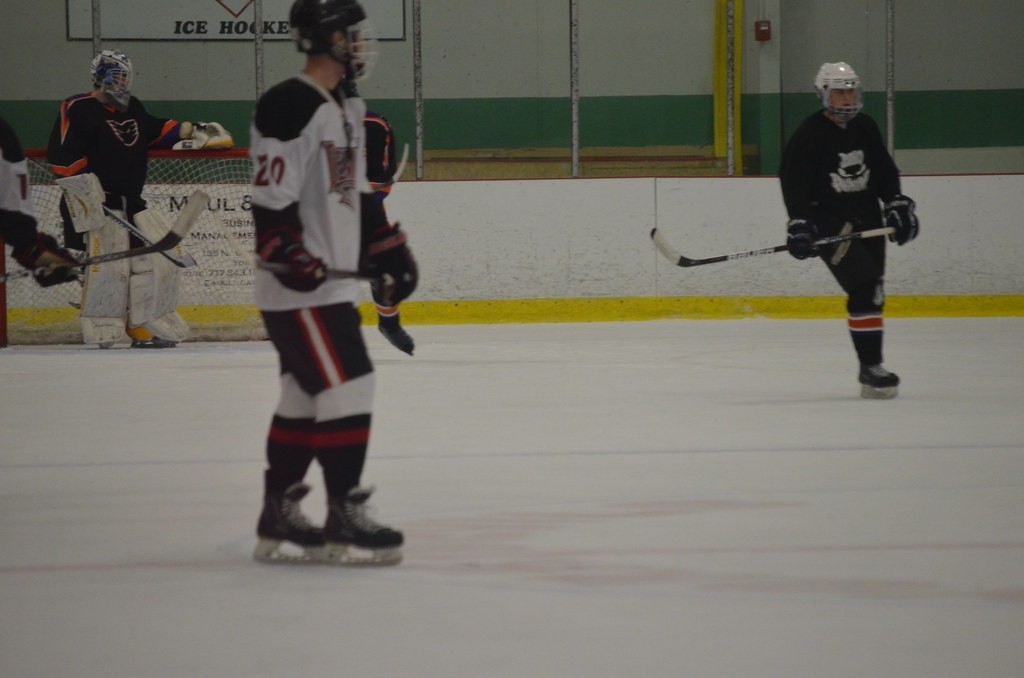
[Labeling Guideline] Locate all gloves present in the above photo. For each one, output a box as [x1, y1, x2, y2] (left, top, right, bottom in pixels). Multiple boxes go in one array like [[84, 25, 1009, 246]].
[[255, 224, 326, 292], [11, 233, 79, 287], [361, 222, 417, 307], [885, 194, 919, 245], [786, 217, 819, 259]]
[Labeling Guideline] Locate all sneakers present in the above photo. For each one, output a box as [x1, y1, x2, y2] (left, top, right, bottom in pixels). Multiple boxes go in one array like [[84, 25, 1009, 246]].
[[858, 364, 899, 399], [323, 487, 404, 561], [252, 468, 326, 558]]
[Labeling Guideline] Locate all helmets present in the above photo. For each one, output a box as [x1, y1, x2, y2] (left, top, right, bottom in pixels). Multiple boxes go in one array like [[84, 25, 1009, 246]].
[[290, 0, 378, 80], [816, 62, 863, 120], [90, 49, 132, 111]]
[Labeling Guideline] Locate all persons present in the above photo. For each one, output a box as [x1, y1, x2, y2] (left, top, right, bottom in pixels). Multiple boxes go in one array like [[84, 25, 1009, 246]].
[[247, 0, 422, 567], [47, 51, 232, 348], [779, 61, 921, 399], [1, 116, 81, 289]]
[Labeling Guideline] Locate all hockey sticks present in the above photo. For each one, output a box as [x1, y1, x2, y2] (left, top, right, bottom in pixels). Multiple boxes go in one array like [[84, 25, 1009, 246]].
[[23, 155, 199, 269], [650, 227, 898, 268], [0, 189, 210, 284], [216, 208, 375, 281]]
